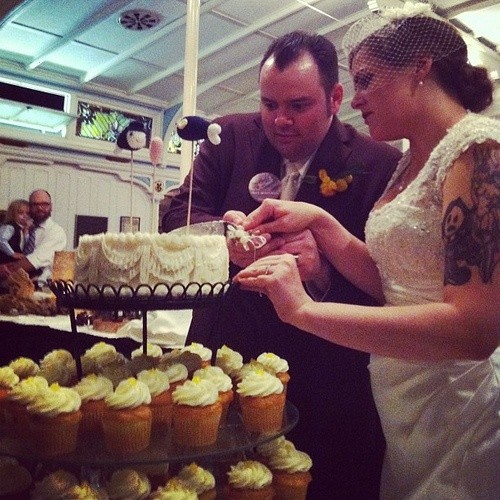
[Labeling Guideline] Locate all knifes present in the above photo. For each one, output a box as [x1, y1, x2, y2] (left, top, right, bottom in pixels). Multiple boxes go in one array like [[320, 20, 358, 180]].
[[168, 219, 251, 239]]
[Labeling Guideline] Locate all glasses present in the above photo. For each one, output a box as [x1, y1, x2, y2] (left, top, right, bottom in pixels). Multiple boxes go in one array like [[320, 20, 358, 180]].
[[32, 202, 50, 206]]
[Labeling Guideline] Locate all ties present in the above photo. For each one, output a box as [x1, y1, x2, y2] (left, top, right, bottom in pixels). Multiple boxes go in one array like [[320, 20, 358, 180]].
[[280, 163, 299, 201], [23, 224, 39, 255]]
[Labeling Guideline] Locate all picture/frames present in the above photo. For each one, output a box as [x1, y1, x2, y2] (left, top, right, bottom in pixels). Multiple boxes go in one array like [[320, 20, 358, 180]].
[[74, 215, 108, 250], [120, 216, 140, 234]]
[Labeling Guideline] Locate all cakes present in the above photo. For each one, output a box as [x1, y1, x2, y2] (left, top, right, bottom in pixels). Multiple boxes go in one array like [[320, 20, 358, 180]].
[[0, 433, 313, 500], [74, 117, 230, 297], [0, 342, 291, 456]]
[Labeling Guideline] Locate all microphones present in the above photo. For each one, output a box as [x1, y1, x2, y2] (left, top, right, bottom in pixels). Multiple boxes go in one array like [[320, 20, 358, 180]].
[[249, 173, 281, 204]]
